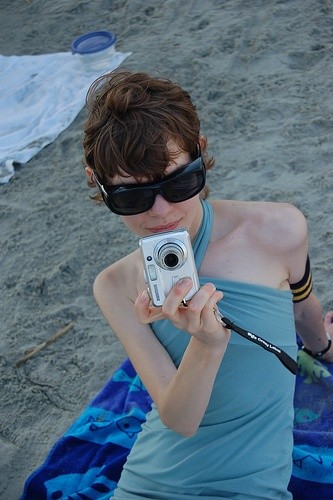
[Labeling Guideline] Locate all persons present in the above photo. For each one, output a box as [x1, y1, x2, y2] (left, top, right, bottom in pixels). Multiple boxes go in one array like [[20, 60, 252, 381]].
[[84, 71, 333, 500]]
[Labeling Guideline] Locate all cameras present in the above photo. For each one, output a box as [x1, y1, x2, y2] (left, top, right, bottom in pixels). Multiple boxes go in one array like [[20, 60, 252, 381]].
[[139, 227, 201, 308]]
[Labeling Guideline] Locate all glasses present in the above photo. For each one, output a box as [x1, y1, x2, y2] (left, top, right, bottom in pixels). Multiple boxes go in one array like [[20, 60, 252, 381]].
[[92, 141, 207, 216]]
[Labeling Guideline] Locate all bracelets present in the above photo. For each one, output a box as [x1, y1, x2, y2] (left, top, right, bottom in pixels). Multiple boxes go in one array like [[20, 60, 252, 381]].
[[302, 339, 332, 356]]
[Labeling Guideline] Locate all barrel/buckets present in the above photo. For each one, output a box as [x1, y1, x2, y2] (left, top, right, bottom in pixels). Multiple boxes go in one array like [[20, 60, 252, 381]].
[[71, 31, 117, 71]]
